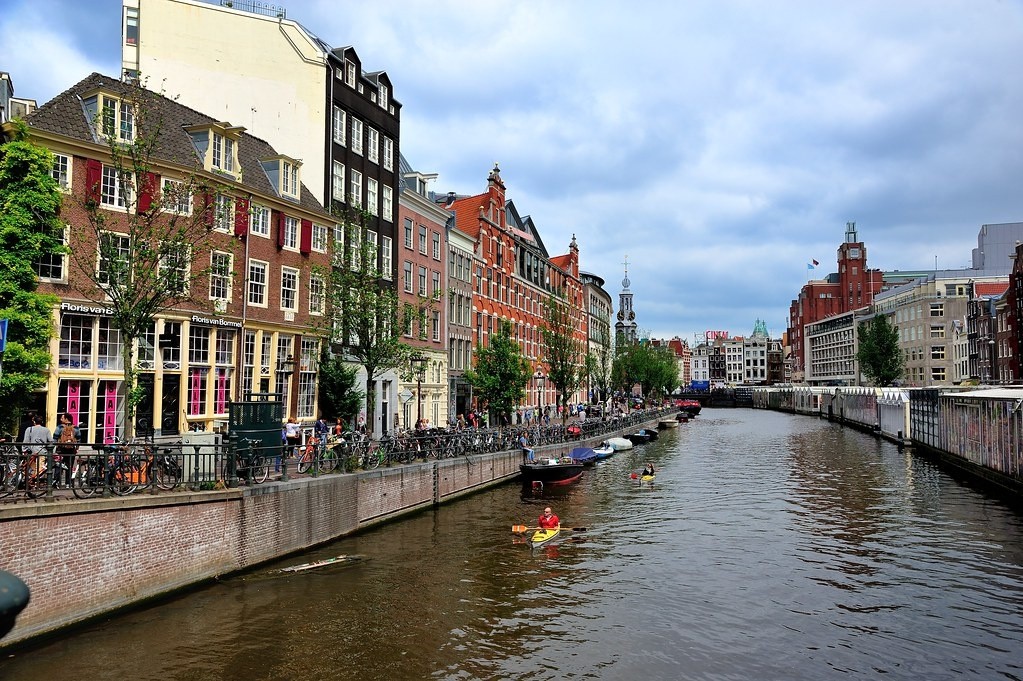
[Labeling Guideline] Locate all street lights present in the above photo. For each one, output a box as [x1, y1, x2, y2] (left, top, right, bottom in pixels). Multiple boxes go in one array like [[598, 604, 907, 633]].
[[411, 355, 431, 426], [534, 365, 546, 427]]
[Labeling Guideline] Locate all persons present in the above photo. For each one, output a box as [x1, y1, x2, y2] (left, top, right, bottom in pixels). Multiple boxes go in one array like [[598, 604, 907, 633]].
[[275, 417, 304, 472], [333, 417, 345, 436], [558, 404, 563, 419], [21, 414, 53, 489], [566, 404, 568, 420], [577, 400, 603, 418], [569, 403, 575, 418], [315, 417, 329, 457], [536, 507, 558, 531], [544, 406, 550, 425], [534, 406, 539, 424], [414, 418, 431, 432], [446, 420, 450, 430], [500, 411, 508, 428], [52, 413, 81, 489], [358, 417, 369, 433], [524, 408, 531, 427], [465, 409, 488, 429], [455, 415, 465, 430], [642, 463, 655, 476], [518, 431, 537, 463], [516, 410, 522, 425]]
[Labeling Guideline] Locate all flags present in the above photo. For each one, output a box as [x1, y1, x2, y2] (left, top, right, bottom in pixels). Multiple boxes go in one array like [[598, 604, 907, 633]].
[[808, 264, 814, 269], [813, 259, 819, 266]]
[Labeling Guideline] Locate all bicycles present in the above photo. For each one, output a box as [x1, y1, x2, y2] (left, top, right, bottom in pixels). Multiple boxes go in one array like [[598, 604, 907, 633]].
[[1, 432, 184, 503], [296, 416, 617, 477], [223, 436, 270, 489]]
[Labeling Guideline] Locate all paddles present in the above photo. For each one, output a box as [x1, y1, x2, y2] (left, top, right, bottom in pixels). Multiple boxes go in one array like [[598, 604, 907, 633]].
[[631, 468, 660, 478], [512, 525, 586, 533]]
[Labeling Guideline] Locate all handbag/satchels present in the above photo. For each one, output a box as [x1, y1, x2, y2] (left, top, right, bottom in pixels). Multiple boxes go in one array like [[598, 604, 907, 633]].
[[286, 432, 295, 437]]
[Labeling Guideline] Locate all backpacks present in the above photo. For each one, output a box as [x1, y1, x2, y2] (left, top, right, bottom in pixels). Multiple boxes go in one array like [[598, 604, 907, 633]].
[[60, 423, 77, 449]]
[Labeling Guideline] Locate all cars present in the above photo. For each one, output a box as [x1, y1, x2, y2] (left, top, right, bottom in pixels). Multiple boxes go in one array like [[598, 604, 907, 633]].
[[584, 404, 602, 417], [630, 397, 644, 404]]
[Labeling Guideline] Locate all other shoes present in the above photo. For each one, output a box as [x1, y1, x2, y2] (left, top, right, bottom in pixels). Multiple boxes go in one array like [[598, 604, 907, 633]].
[[66, 484, 72, 488]]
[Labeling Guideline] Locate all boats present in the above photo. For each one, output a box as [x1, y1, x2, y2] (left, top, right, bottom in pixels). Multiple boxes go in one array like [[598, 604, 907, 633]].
[[593, 445, 615, 459], [569, 448, 599, 467], [658, 420, 679, 428], [623, 433, 650, 442], [519, 456, 583, 494], [603, 437, 633, 450], [640, 474, 656, 484], [680, 399, 702, 414], [675, 413, 689, 422], [639, 429, 659, 441], [530, 524, 560, 548]]
